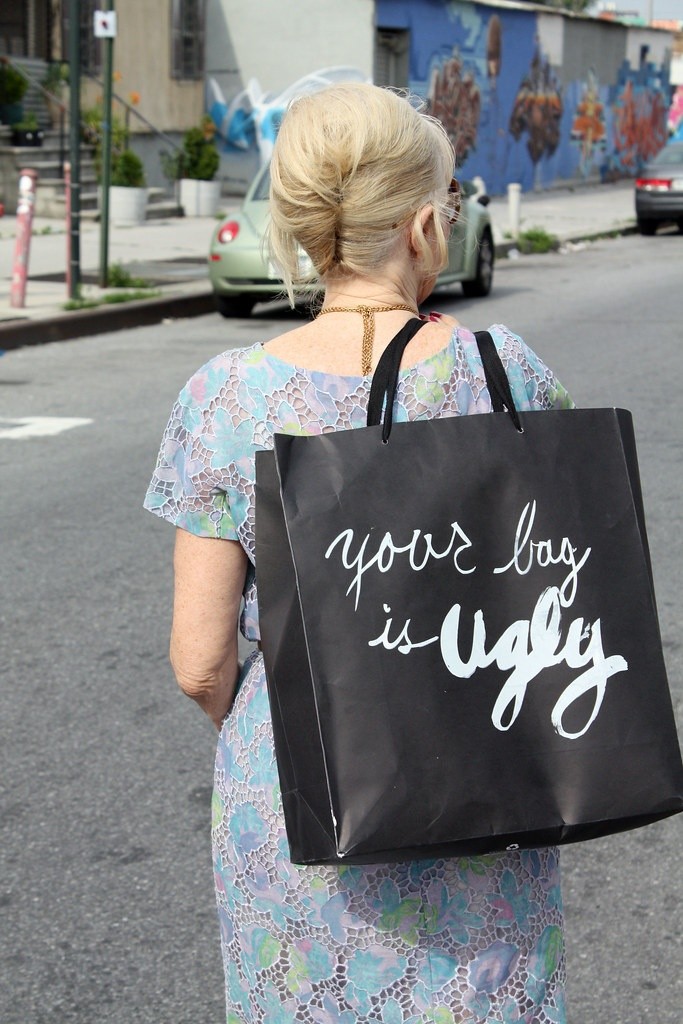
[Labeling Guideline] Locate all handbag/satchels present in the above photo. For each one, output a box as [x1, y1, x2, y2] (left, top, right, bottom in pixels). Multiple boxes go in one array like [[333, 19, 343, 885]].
[[255, 319, 682, 863]]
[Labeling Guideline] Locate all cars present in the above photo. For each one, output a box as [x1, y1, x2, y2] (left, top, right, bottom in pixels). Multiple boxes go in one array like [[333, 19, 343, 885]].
[[208, 150, 497, 320], [635, 142, 683, 235]]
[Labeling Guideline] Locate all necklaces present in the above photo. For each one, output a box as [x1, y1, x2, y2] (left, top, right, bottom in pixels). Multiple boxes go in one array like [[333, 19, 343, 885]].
[[315, 304, 419, 376]]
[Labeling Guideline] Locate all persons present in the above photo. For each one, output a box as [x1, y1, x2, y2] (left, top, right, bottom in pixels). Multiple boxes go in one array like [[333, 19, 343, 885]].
[[144, 84, 574, 1024]]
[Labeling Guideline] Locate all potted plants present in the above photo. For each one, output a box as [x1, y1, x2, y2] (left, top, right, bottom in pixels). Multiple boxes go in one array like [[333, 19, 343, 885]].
[[41, 63, 69, 128], [164, 125, 221, 216], [82, 101, 150, 225], [14, 115, 43, 146], [0, 66, 28, 125]]
